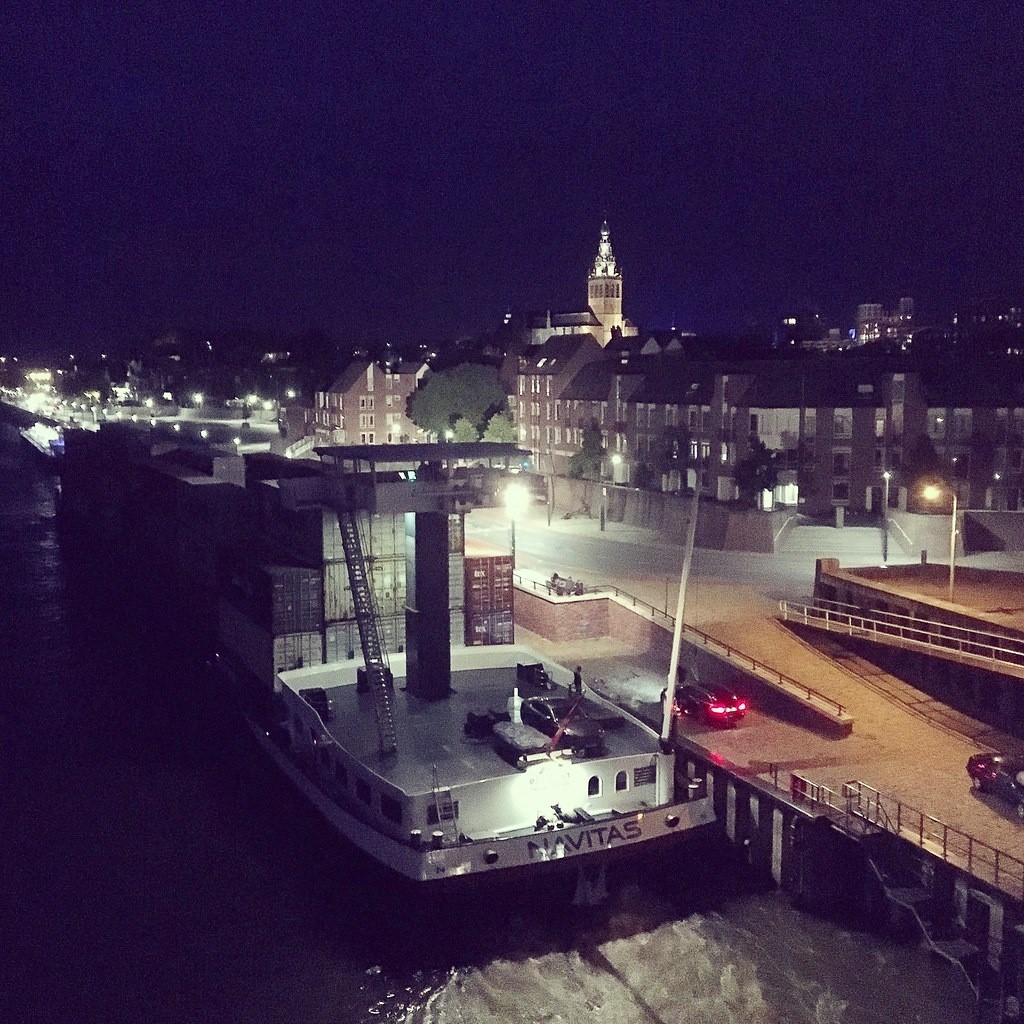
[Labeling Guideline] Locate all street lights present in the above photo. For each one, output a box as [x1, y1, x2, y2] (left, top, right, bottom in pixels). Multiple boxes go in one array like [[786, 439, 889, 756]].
[[505, 483, 527, 568], [923, 486, 957, 602], [883, 472, 891, 565]]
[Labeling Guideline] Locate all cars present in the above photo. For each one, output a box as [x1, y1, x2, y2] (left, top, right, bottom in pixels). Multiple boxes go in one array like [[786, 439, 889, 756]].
[[661, 681, 745, 724], [965, 753, 1024, 818], [521, 696, 606, 751]]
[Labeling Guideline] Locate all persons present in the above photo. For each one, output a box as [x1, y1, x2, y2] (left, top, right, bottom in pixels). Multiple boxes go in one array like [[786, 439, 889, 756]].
[[573, 664, 582, 695], [574, 580, 583, 595], [565, 576, 575, 594], [552, 572, 560, 588], [557, 579, 566, 596]]
